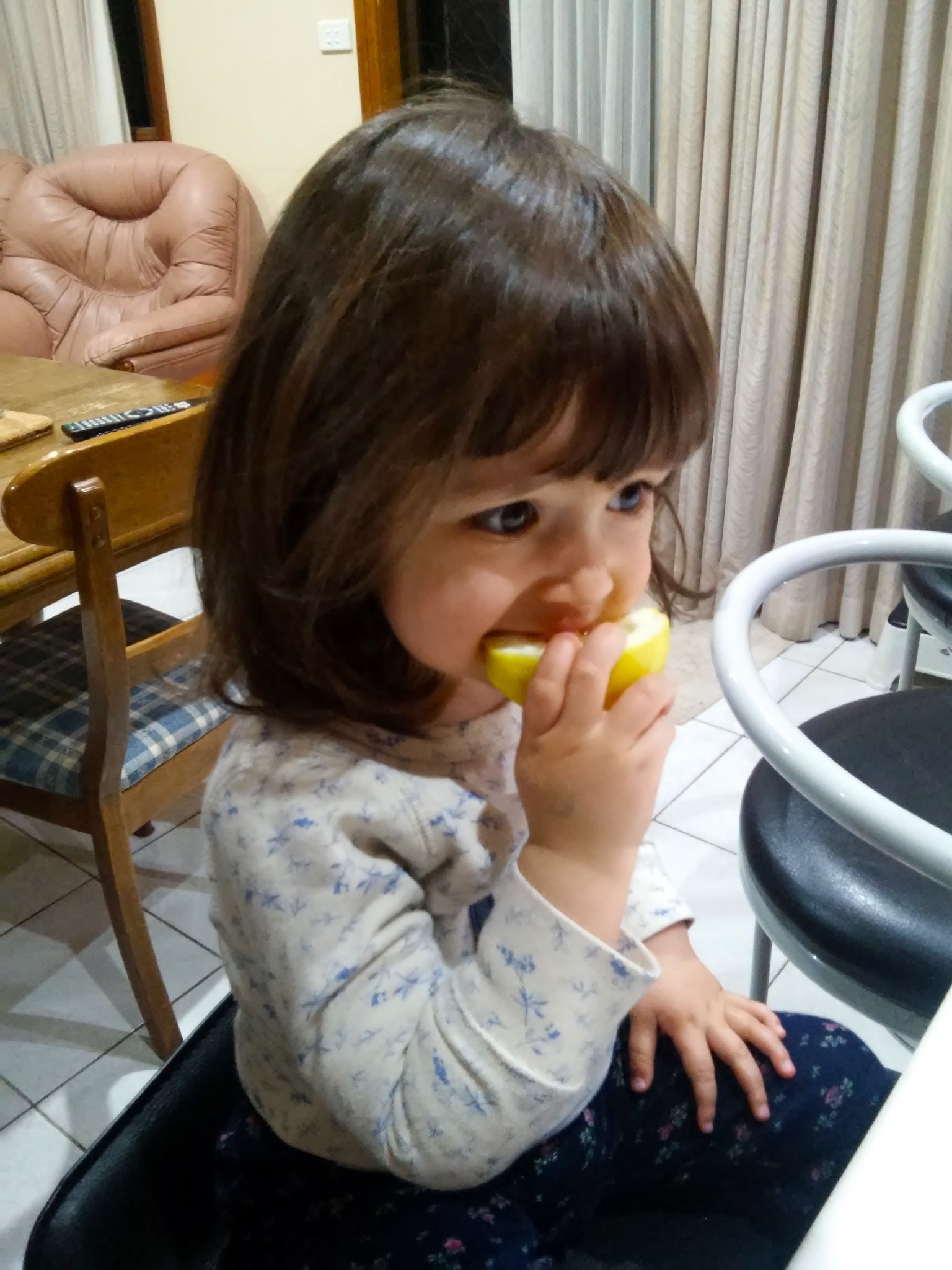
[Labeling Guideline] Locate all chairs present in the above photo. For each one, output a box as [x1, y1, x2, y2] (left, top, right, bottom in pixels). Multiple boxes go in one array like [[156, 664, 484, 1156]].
[[0, 403, 252, 1062], [711, 529, 952, 1052], [896, 381, 952, 692]]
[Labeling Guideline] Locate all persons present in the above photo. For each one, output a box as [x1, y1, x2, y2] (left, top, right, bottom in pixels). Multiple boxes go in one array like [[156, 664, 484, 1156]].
[[22, 93, 901, 1270]]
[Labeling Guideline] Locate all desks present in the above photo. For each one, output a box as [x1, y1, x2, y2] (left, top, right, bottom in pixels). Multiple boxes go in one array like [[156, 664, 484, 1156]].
[[0, 352, 218, 640]]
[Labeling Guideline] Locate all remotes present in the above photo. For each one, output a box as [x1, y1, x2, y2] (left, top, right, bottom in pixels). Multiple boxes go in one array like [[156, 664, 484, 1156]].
[[61, 395, 212, 442]]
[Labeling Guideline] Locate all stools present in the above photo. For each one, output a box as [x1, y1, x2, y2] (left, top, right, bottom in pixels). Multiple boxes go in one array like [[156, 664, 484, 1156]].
[[865, 599, 952, 692]]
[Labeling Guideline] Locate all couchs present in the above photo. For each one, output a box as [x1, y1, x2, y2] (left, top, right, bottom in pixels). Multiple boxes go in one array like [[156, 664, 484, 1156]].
[[0, 142, 268, 387]]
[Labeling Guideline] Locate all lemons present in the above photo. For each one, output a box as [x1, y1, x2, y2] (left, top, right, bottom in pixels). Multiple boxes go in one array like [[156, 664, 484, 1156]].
[[482, 606, 671, 710]]
